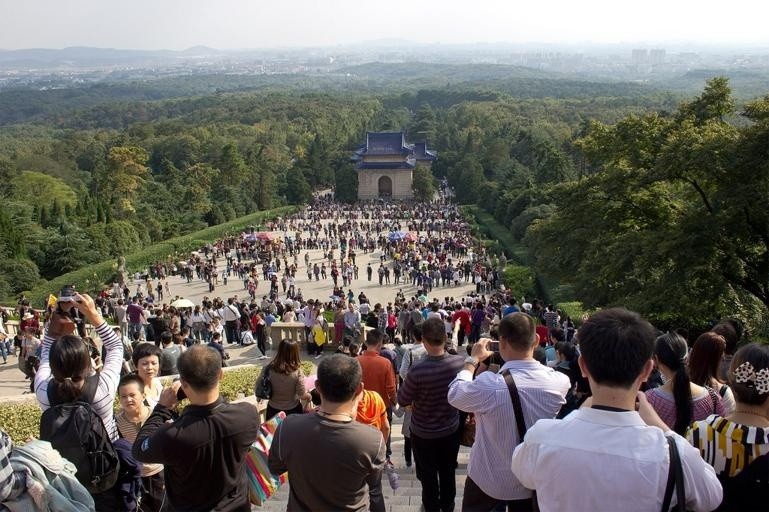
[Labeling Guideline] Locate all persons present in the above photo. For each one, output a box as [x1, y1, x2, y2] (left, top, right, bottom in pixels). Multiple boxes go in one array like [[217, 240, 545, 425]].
[[0, 182, 769, 512]]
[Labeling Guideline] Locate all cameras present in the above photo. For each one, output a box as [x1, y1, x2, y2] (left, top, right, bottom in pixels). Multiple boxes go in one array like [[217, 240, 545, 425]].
[[488, 341, 501, 352], [58, 284, 83, 303], [177, 387, 188, 400]]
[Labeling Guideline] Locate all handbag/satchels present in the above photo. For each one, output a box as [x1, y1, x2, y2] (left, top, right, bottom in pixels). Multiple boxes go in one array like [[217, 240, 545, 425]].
[[460, 420, 475, 447], [246, 410, 288, 506], [255, 375, 272, 400]]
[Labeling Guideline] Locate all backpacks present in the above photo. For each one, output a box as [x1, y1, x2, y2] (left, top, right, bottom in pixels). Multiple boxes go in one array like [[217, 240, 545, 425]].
[[40, 376, 120, 494]]
